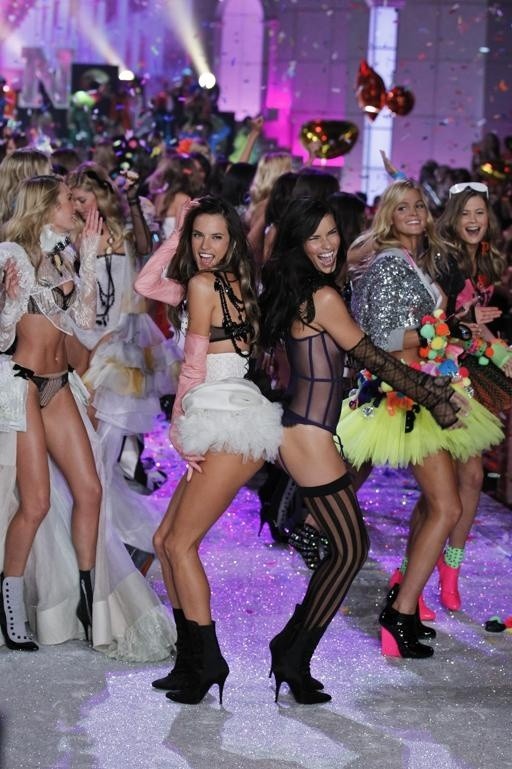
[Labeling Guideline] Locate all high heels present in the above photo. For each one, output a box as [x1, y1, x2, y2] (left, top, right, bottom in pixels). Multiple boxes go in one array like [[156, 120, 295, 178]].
[[166, 619, 230, 706], [390, 567, 436, 620], [151, 609, 189, 689], [270, 625, 333, 705], [380, 583, 436, 639], [0, 572, 40, 652], [436, 555, 461, 611], [75, 567, 95, 642], [379, 605, 434, 660], [267, 604, 325, 690]]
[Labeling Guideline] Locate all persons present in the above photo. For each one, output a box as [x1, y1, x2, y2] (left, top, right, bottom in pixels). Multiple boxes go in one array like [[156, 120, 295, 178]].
[[268, 196, 473, 706], [154, 155, 196, 239], [264, 171, 296, 261], [381, 149, 432, 186], [0, 148, 161, 580], [133, 195, 282, 706], [483, 133, 498, 161], [336, 180, 502, 659], [290, 141, 322, 172], [245, 151, 292, 249], [210, 115, 262, 192], [224, 163, 251, 216], [67, 163, 184, 433], [6, 134, 28, 154], [387, 181, 512, 621], [1, 177, 177, 665], [92, 141, 116, 169], [188, 151, 211, 183]]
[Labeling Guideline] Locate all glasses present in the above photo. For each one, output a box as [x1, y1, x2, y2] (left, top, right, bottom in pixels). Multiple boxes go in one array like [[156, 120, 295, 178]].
[[447, 181, 490, 201]]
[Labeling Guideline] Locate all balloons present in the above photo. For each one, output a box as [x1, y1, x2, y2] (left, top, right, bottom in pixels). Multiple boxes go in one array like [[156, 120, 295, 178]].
[[297, 118, 359, 158], [385, 86, 416, 116], [354, 61, 385, 126]]
